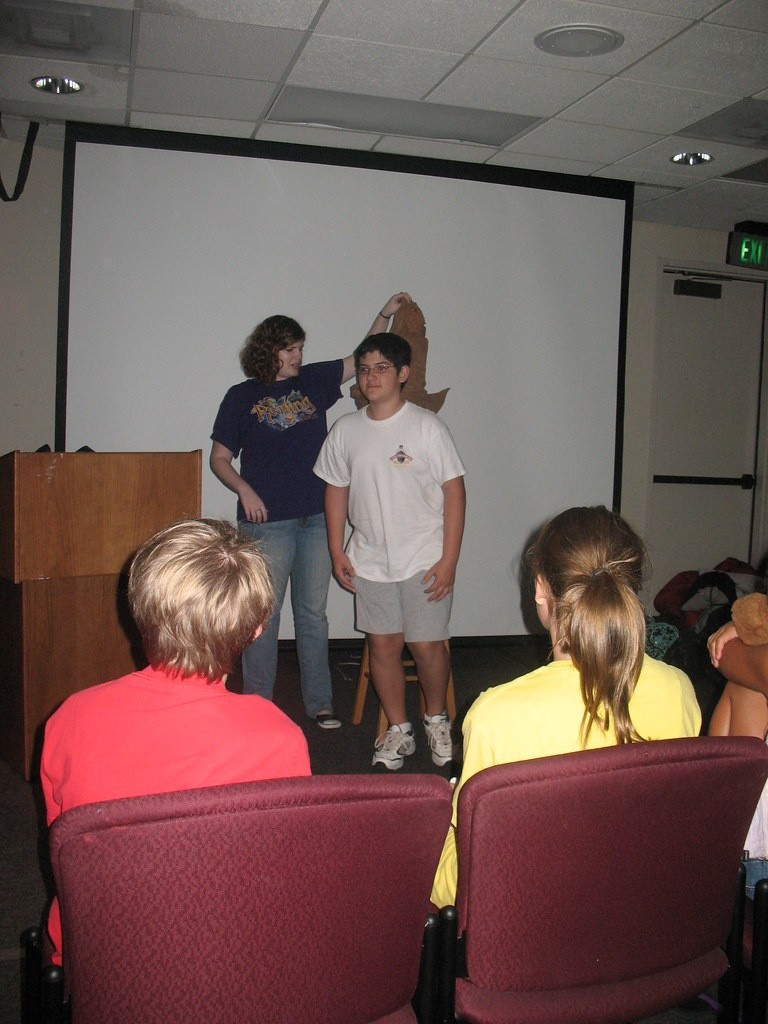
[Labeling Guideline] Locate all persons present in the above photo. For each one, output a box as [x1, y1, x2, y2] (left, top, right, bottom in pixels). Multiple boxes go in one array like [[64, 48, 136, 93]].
[[431, 504, 702, 911], [210, 291, 414, 729], [38, 520, 311, 967], [313, 333, 468, 769], [706, 555, 768, 862]]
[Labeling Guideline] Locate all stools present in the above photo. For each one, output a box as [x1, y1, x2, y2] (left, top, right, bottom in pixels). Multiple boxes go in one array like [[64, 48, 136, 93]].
[[350, 636, 454, 734]]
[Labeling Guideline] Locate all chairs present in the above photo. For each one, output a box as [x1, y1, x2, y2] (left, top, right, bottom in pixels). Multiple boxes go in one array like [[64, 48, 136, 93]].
[[19, 735, 768, 1024]]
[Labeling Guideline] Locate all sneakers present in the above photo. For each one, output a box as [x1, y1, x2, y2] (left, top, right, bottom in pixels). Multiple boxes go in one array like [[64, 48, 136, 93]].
[[372, 725, 416, 772], [316, 713, 341, 729], [422, 713, 460, 767]]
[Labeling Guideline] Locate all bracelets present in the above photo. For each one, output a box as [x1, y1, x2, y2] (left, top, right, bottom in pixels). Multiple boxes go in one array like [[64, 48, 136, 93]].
[[379, 310, 390, 319]]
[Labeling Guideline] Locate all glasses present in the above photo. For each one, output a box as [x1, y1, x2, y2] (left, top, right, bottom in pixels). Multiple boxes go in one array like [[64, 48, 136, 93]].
[[354, 362, 396, 374]]
